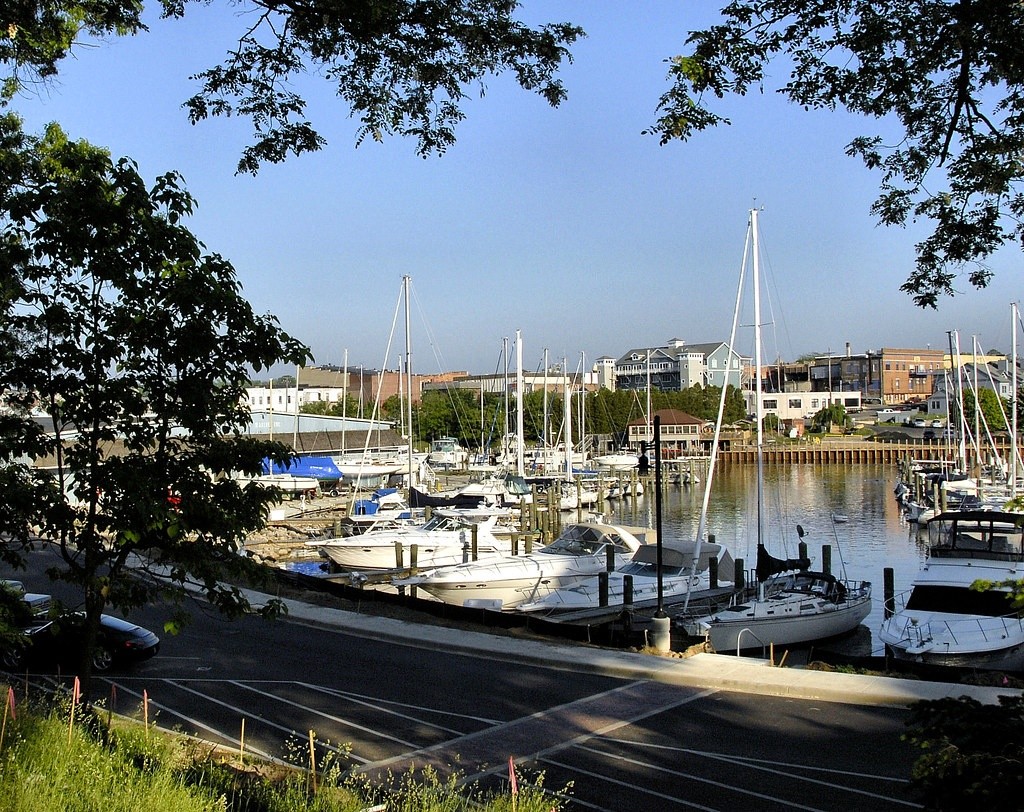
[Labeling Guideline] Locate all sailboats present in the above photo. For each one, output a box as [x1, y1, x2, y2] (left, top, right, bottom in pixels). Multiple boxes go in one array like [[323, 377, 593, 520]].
[[633, 193, 874, 654], [165, 271, 1024, 630]]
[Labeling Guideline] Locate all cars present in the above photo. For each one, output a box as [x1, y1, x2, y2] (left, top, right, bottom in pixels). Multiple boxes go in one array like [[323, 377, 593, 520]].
[[1, 620, 52, 670], [70, 610, 162, 674]]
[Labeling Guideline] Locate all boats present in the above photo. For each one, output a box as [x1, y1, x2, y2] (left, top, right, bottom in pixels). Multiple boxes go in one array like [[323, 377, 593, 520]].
[[877, 585, 1024, 679]]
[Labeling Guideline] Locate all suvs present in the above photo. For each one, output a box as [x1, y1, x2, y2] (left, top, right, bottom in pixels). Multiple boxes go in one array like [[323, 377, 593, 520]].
[[0, 578, 53, 619]]
